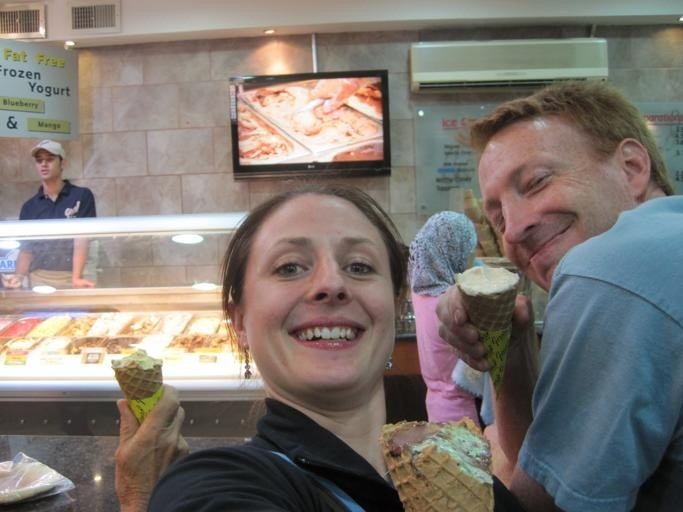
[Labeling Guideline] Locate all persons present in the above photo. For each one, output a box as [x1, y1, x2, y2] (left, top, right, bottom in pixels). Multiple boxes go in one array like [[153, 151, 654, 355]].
[[0, 138, 97, 289], [433, 77, 682, 512], [406, 210, 484, 436], [114, 387, 193, 511], [144, 183, 529, 512]]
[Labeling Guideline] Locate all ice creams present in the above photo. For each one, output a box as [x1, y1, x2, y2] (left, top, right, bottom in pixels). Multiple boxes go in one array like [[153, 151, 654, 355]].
[[110, 348, 164, 424], [454, 264, 520, 396], [376, 415, 494, 512]]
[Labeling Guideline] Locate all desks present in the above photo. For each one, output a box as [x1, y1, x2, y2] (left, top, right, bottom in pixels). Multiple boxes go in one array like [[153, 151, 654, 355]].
[[0, 435, 261, 512]]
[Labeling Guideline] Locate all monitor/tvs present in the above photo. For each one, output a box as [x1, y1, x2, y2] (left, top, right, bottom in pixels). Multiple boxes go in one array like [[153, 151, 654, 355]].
[[228, 69, 392, 180]]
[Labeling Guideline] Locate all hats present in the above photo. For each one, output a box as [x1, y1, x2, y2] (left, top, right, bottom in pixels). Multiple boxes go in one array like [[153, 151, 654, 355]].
[[31, 140, 65, 159]]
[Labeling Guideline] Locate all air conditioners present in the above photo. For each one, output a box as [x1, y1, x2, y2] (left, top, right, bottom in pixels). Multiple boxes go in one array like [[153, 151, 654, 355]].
[[409, 38, 608, 94]]
